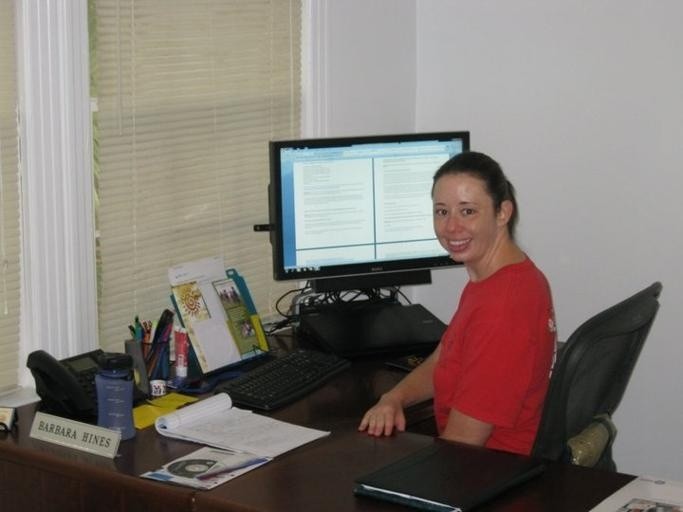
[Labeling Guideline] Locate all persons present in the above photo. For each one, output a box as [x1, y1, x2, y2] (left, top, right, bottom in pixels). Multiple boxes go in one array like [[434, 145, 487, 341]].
[[357, 149, 559, 455]]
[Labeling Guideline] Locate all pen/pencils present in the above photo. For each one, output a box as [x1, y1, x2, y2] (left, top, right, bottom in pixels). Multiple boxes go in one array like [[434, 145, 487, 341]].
[[128, 310, 173, 381]]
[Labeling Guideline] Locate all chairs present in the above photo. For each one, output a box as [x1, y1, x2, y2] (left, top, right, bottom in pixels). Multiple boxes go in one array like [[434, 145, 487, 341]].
[[528, 272, 662, 474]]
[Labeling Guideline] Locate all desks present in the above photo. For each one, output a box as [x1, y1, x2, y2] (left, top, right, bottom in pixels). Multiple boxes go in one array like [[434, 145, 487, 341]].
[[0, 326, 637, 512]]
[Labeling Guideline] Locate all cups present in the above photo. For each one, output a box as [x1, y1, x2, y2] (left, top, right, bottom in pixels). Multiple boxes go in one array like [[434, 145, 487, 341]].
[[94, 351, 137, 439]]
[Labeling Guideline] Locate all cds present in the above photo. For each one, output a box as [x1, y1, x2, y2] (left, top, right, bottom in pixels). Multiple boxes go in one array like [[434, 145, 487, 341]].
[[167, 459, 219, 479]]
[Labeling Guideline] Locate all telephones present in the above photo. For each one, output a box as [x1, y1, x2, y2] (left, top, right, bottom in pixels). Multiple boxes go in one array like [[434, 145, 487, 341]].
[[26, 350, 105, 425]]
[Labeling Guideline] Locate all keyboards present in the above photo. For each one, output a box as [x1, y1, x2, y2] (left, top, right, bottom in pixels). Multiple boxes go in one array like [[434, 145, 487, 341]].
[[214, 348, 353, 411]]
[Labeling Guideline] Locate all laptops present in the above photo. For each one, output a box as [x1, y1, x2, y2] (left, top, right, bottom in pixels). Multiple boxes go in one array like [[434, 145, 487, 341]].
[[301, 303, 448, 358]]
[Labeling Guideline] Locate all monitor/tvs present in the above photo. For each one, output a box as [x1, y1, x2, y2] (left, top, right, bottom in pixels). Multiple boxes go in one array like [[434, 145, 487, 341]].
[[269, 130, 471, 281]]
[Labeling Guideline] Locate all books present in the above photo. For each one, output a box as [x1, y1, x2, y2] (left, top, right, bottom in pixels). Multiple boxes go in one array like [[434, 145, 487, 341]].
[[347, 438, 554, 511], [153, 391, 333, 458]]
